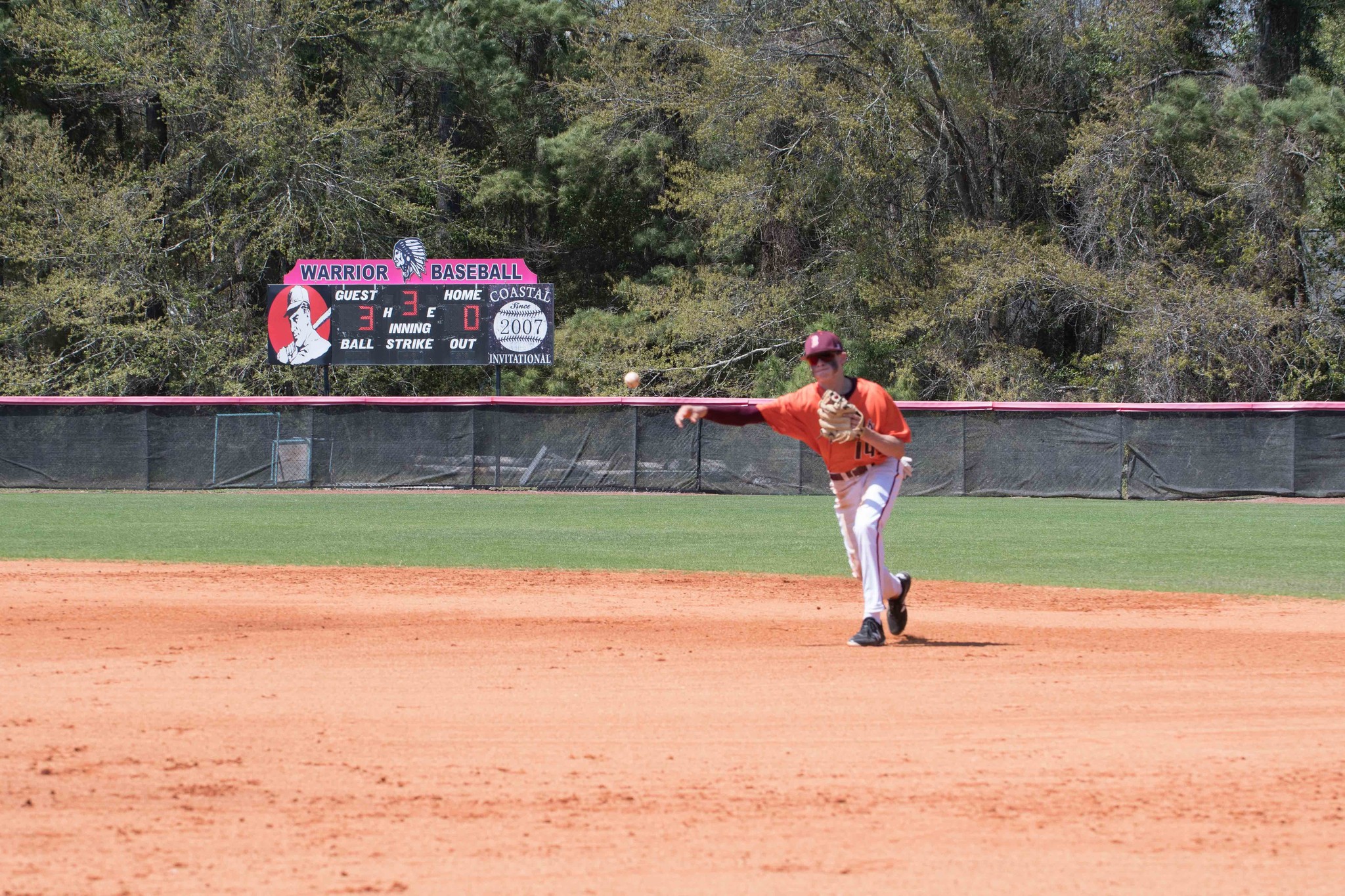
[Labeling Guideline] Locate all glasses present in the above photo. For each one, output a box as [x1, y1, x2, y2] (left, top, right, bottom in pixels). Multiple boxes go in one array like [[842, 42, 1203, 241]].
[[807, 352, 841, 366]]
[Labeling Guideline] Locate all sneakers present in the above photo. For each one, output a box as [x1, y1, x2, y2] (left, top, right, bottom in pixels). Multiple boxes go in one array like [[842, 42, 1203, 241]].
[[846, 617, 886, 646], [886, 571, 911, 635]]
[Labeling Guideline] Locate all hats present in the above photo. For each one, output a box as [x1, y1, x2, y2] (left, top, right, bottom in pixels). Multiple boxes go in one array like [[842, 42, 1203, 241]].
[[802, 331, 842, 360]]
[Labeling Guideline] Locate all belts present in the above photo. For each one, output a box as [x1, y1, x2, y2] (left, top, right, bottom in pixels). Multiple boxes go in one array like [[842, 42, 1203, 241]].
[[828, 464, 876, 480]]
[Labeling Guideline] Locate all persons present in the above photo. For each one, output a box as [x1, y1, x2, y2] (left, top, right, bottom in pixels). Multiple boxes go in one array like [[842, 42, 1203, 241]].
[[674, 331, 914, 647]]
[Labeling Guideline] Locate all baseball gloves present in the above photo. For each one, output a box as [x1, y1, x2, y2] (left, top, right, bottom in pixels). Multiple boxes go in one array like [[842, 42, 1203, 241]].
[[816, 389, 865, 444]]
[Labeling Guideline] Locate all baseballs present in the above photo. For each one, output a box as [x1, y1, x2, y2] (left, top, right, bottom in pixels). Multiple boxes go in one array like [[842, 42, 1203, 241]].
[[624, 372, 641, 389]]
